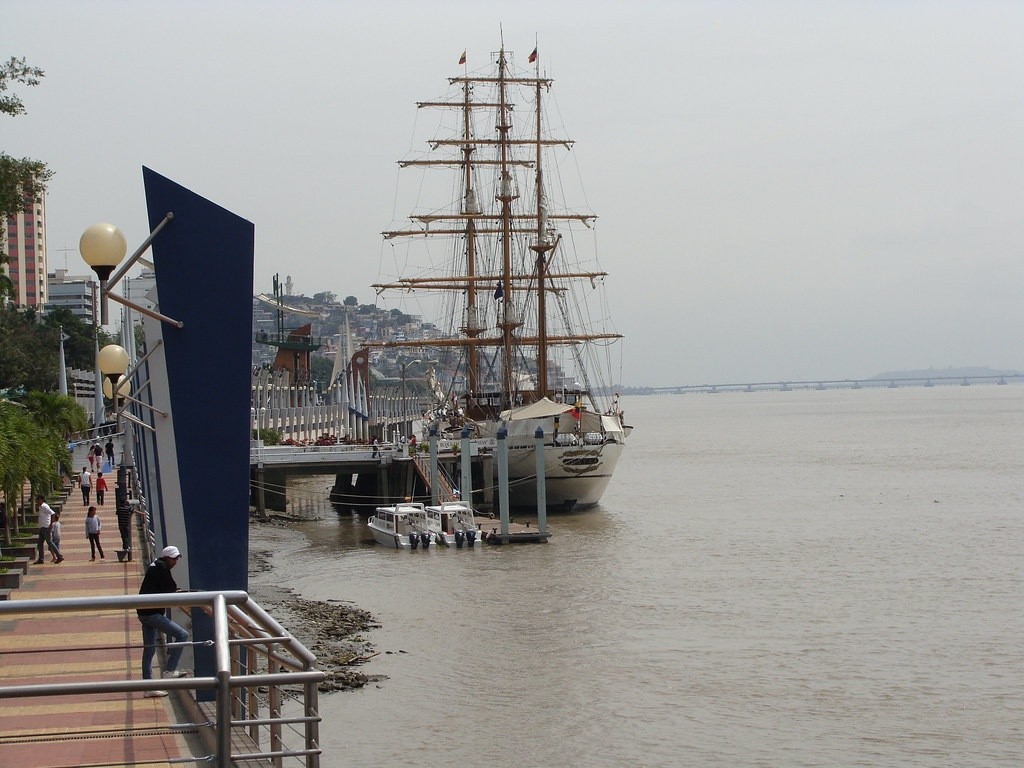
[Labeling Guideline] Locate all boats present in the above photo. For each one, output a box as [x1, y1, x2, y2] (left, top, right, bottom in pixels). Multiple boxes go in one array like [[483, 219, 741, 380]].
[[367, 502, 441, 550], [418, 501, 483, 547]]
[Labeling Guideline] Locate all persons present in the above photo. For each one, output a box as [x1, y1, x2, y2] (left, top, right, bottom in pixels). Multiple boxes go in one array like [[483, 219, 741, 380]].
[[401, 434, 405, 444], [374, 437, 382, 458], [84, 505, 104, 561], [86, 443, 104, 473], [96, 472, 108, 506], [410, 435, 417, 449], [136, 545, 188, 698], [32, 495, 64, 565], [105, 438, 115, 468], [115, 496, 147, 549], [78, 466, 93, 506], [450, 492, 459, 502]]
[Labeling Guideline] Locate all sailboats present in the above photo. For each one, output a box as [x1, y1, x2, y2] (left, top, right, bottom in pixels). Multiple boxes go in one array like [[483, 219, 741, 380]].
[[352, 17, 635, 518]]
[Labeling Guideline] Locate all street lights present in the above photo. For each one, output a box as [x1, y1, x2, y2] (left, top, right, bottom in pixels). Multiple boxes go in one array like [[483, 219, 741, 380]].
[[401, 360, 439, 458]]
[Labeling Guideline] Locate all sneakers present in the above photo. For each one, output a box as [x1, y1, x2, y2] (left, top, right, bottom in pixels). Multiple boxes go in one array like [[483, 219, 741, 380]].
[[162, 670, 187, 679], [143, 690, 169, 698]]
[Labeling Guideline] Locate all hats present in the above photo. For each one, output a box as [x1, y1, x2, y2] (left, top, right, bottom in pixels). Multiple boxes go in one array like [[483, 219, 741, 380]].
[[162, 546, 183, 558], [94, 443, 99, 445]]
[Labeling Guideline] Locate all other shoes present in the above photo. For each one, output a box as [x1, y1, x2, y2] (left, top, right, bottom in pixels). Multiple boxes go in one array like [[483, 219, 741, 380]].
[[55, 556, 64, 564], [89, 559, 95, 561], [33, 559, 44, 564], [50, 559, 56, 563]]
